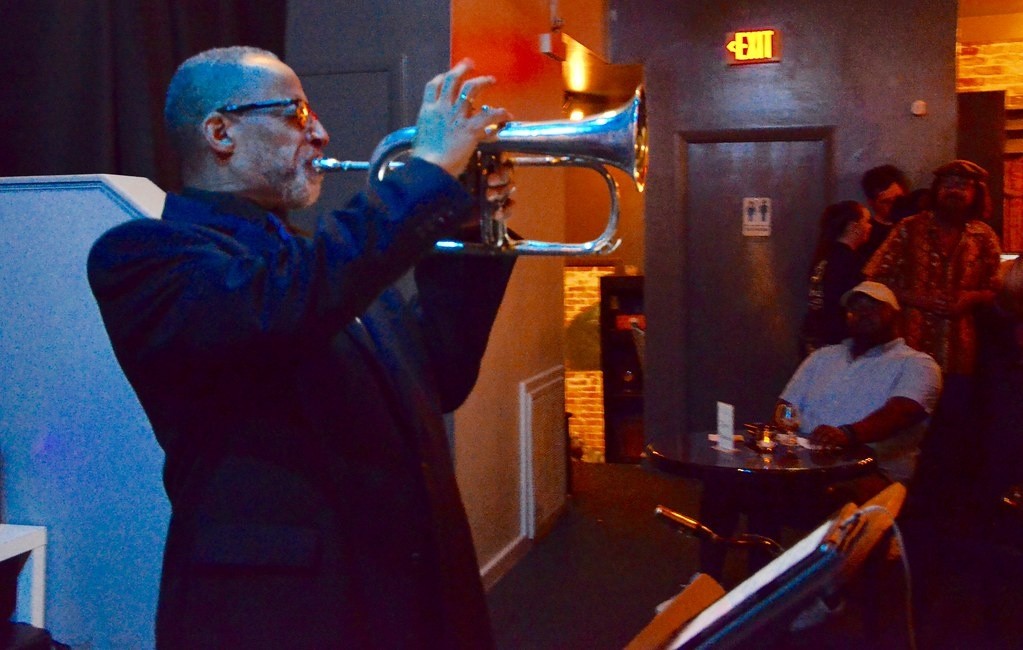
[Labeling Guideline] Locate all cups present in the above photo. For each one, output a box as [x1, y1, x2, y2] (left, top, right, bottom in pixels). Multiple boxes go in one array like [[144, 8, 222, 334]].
[[754, 426, 778, 451]]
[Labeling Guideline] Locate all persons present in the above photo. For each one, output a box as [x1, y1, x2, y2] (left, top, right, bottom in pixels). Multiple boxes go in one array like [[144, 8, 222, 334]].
[[895, 187, 929, 224], [859, 163, 912, 267], [862, 160, 1005, 594], [657, 280, 942, 631], [89, 45, 524, 650], [802, 200, 873, 355]]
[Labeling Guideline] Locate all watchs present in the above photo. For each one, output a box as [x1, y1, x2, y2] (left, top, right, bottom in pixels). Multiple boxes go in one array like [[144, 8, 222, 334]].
[[838, 424, 856, 439]]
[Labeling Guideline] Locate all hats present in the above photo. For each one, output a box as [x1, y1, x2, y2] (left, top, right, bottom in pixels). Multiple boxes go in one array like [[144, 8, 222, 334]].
[[839, 281, 901, 312], [933, 160, 992, 181]]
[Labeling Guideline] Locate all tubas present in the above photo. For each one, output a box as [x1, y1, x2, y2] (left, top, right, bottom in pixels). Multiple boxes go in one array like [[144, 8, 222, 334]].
[[304, 78, 649, 257]]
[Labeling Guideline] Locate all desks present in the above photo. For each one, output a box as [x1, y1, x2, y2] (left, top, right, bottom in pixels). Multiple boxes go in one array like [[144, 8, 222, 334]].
[[643, 429, 875, 581]]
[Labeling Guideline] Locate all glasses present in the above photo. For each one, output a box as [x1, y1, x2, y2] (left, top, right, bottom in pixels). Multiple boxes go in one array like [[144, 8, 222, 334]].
[[940, 181, 972, 192], [198, 97, 318, 136]]
